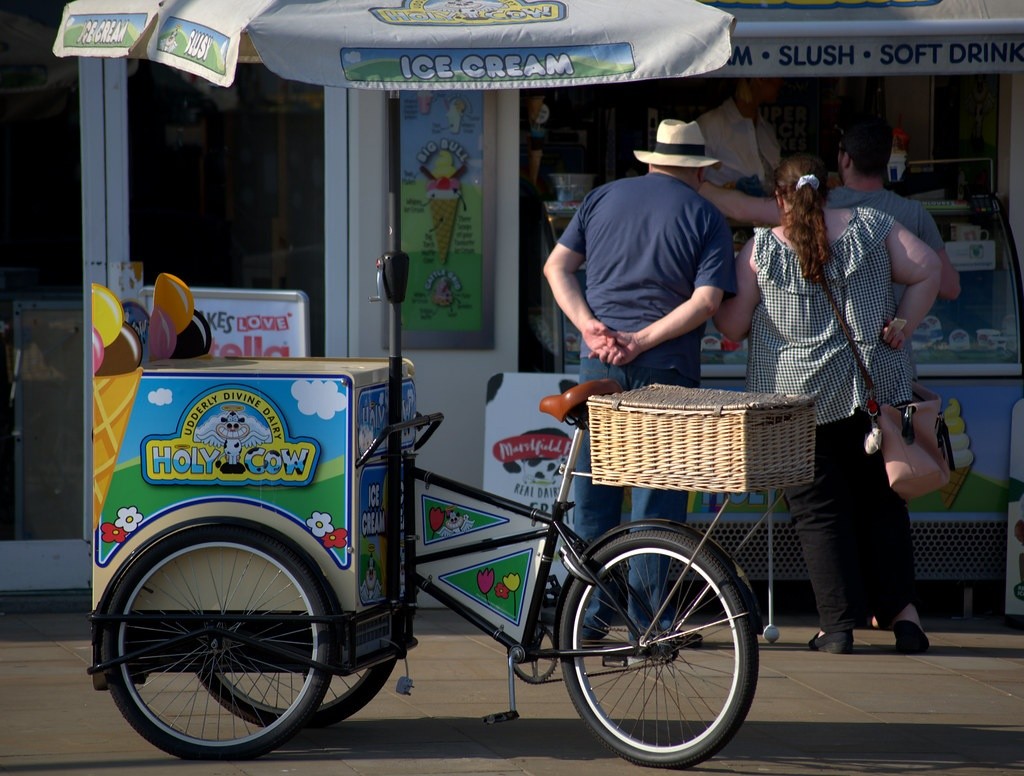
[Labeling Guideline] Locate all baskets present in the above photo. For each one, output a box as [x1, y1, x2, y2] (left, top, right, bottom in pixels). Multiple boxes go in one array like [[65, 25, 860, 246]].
[[586, 383, 817, 491]]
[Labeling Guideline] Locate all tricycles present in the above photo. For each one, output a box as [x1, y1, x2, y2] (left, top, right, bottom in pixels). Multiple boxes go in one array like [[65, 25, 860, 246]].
[[86, 250, 818, 771]]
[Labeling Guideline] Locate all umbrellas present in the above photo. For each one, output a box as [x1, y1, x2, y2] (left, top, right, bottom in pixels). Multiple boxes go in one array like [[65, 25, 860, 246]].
[[50, 1, 736, 602]]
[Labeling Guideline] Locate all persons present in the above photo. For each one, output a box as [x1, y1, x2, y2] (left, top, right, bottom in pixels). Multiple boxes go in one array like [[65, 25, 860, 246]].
[[698, 111, 961, 628], [542, 119, 735, 645], [696, 77, 782, 185], [712, 154, 944, 654]]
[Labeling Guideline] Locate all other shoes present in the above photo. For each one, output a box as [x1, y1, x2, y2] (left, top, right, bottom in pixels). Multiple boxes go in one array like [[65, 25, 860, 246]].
[[894, 620, 929, 653], [808, 630, 853, 653], [669, 625, 703, 648], [581, 638, 603, 649]]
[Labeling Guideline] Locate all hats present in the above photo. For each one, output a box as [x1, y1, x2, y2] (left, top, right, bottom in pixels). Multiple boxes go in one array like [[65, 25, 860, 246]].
[[633, 119, 722, 170]]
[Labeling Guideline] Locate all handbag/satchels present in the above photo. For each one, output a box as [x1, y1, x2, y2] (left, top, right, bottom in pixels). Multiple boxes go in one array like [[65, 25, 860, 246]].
[[868, 380, 955, 500]]
